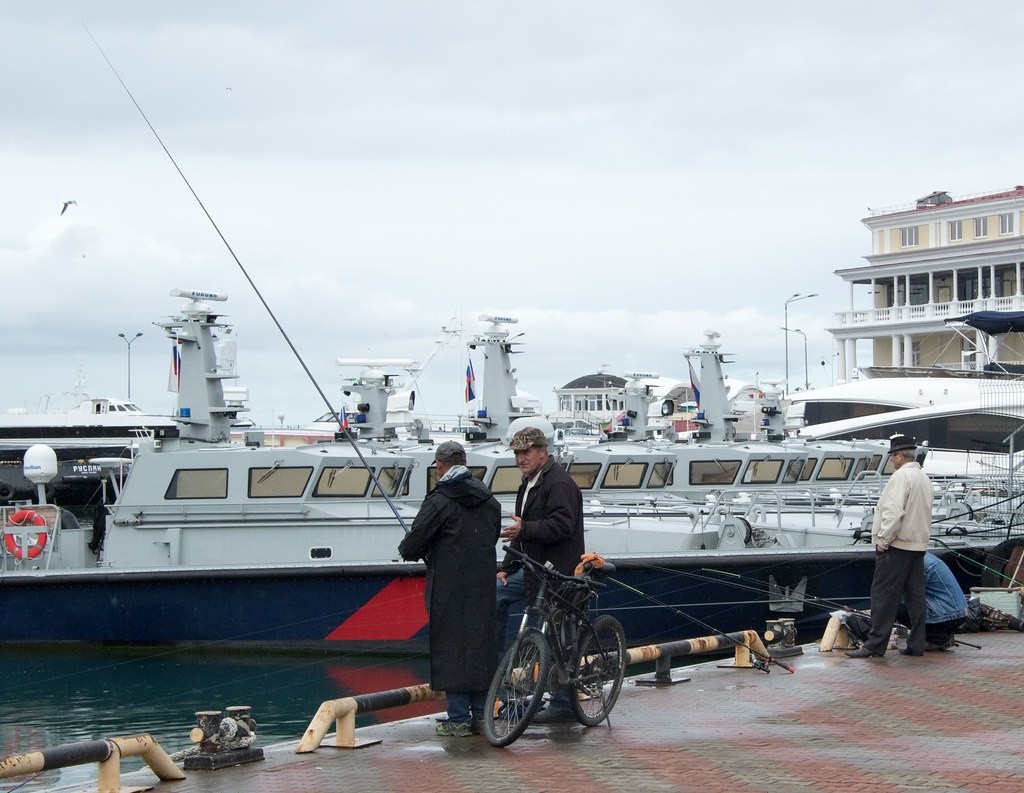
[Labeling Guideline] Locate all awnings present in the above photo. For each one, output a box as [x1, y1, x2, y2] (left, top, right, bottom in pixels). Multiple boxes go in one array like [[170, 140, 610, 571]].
[[884, 310, 1024, 380]]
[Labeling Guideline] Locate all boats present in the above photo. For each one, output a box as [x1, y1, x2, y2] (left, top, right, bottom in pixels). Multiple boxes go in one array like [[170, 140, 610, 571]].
[[0, 396, 190, 525], [0, 280, 1024, 661]]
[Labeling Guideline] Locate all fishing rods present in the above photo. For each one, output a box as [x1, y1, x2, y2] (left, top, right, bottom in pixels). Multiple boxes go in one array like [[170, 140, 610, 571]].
[[81, 20, 435, 575], [601, 542, 1024, 651], [608, 574, 796, 675]]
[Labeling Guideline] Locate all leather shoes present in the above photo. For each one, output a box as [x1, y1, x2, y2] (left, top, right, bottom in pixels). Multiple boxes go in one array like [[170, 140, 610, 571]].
[[897, 647, 924, 656], [844, 647, 884, 657]]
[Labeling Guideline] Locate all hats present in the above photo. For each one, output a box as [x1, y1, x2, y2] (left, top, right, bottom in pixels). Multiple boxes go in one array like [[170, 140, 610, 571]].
[[505, 426, 548, 452], [431, 440, 466, 465], [887, 436, 917, 454]]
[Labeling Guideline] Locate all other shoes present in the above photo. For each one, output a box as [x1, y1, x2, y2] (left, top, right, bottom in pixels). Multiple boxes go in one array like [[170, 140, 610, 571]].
[[530, 706, 579, 723], [924, 633, 955, 652]]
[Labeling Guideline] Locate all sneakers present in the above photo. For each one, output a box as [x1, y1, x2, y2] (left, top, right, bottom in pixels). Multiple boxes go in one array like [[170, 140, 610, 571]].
[[435, 718, 486, 737]]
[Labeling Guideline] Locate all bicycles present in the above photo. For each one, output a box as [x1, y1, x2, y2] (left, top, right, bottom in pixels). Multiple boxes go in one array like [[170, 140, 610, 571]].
[[483, 544, 627, 748]]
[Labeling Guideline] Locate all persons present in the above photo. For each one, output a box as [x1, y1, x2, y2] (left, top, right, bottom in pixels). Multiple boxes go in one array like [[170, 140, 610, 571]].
[[399, 425, 501, 738], [843, 435, 935, 658], [894, 550, 971, 653], [498, 427, 586, 728]]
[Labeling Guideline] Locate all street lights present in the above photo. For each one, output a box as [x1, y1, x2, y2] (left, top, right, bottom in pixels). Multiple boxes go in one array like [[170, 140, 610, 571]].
[[117, 332, 144, 402], [785, 290, 819, 398], [781, 327, 809, 390]]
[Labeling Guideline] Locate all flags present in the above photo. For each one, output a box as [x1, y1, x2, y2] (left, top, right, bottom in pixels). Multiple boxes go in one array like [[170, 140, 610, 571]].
[[463, 344, 479, 404], [685, 357, 703, 411], [336, 402, 351, 436], [165, 332, 183, 396]]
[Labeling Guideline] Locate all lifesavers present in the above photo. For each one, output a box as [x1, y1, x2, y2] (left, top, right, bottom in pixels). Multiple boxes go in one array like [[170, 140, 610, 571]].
[[2, 509, 48, 561]]
